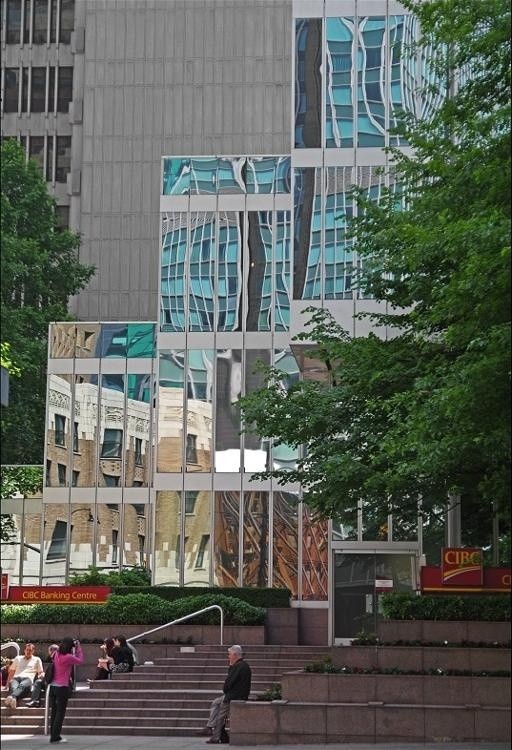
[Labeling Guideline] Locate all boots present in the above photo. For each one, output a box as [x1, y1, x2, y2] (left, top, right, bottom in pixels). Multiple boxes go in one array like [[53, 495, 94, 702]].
[[86, 667, 108, 685]]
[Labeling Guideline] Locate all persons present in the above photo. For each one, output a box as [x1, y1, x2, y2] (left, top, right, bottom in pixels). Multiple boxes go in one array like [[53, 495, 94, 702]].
[[193, 644, 252, 744], [2, 635, 139, 744]]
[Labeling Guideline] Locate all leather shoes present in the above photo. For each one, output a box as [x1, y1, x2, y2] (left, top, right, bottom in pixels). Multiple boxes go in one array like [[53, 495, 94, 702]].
[[24, 701, 40, 707]]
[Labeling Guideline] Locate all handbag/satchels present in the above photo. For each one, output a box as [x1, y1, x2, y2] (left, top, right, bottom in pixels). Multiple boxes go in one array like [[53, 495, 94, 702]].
[[220, 724, 229, 743], [46, 663, 54, 684]]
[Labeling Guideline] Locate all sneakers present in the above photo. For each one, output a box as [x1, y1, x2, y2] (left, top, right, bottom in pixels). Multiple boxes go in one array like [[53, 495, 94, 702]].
[[194, 727, 219, 743], [52, 739, 67, 744], [5, 696, 17, 710]]
